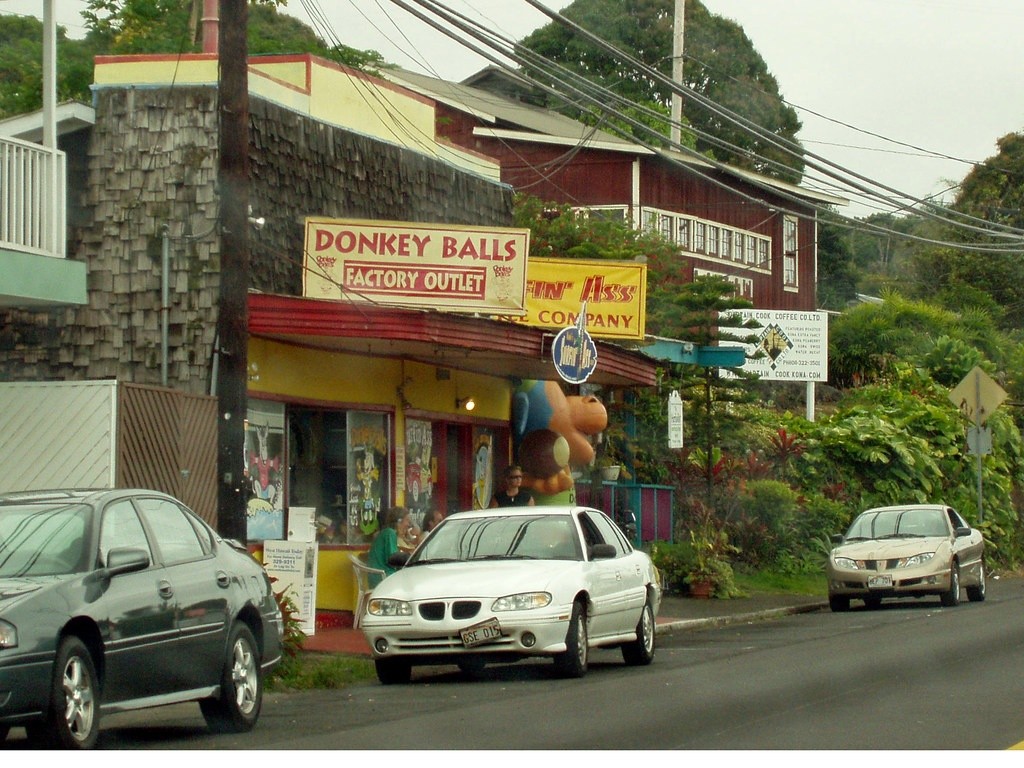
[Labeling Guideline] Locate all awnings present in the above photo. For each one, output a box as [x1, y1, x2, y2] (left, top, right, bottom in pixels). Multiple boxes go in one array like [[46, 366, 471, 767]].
[[601, 332, 747, 366]]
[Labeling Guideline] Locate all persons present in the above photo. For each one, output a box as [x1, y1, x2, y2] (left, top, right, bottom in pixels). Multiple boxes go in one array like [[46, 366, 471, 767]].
[[366, 506, 442, 590], [488, 465, 535, 509]]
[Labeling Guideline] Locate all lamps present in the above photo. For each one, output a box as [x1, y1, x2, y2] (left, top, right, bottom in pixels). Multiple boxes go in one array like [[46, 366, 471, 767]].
[[248, 217, 265, 230], [249, 373, 259, 383], [456, 396, 475, 410]]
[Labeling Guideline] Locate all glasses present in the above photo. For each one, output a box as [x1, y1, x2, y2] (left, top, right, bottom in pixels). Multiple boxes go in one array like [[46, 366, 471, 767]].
[[508, 475, 522, 479]]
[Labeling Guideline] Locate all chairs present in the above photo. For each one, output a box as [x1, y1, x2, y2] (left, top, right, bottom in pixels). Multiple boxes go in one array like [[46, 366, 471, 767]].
[[340, 542, 387, 630]]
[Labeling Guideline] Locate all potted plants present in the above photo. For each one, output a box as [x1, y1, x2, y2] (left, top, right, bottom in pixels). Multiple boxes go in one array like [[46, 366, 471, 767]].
[[594, 428, 626, 481], [644, 520, 751, 598]]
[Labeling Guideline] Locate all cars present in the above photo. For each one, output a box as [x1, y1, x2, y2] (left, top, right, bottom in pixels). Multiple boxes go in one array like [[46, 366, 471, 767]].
[[827, 504, 986, 612], [362, 506, 661, 685], [0, 489, 284, 750]]
